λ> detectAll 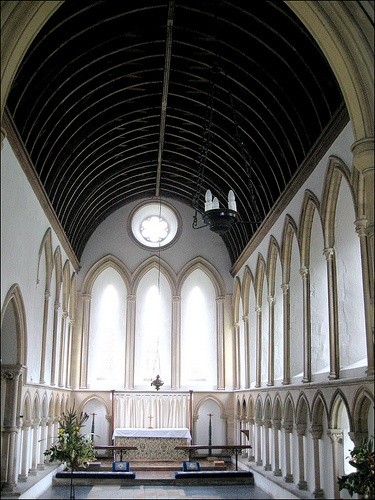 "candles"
[212,197,220,210]
[227,189,237,209]
[203,190,212,210]
